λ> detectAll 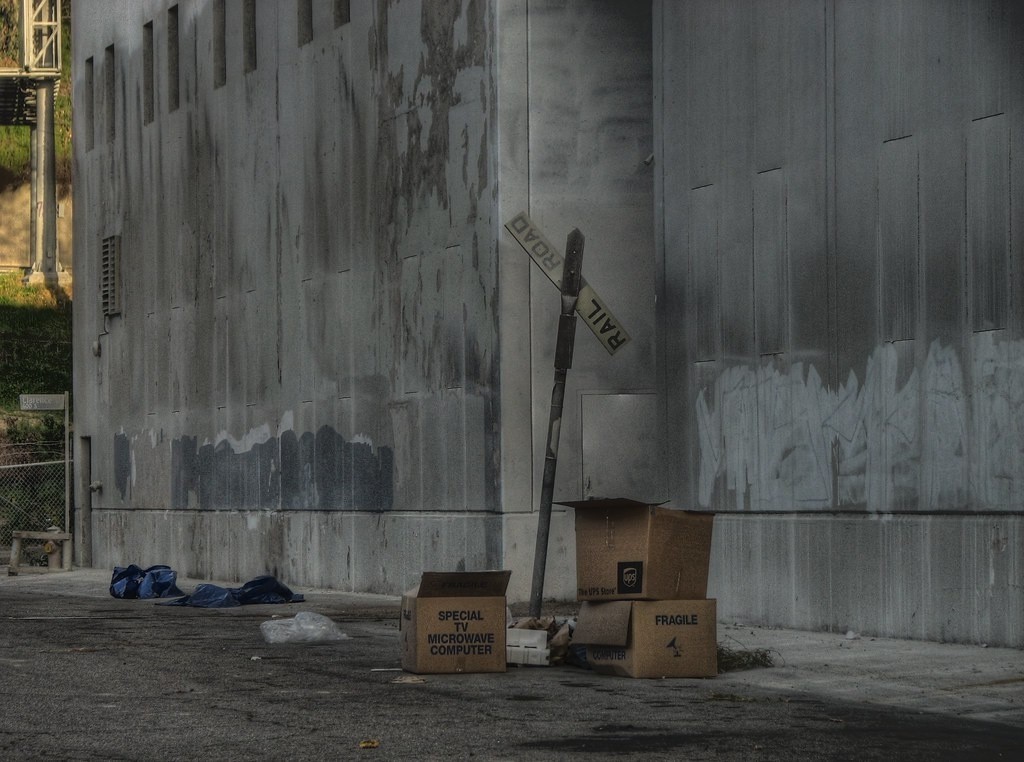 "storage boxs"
[567,598,719,678]
[550,497,716,600]
[398,571,513,673]
